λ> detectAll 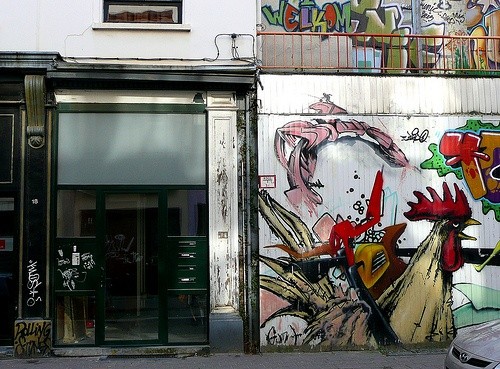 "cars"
[442,319,500,368]
